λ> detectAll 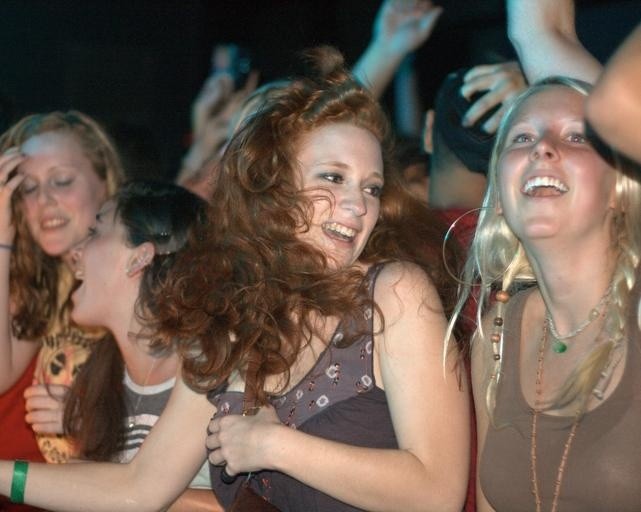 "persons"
[0,1,641,512]
